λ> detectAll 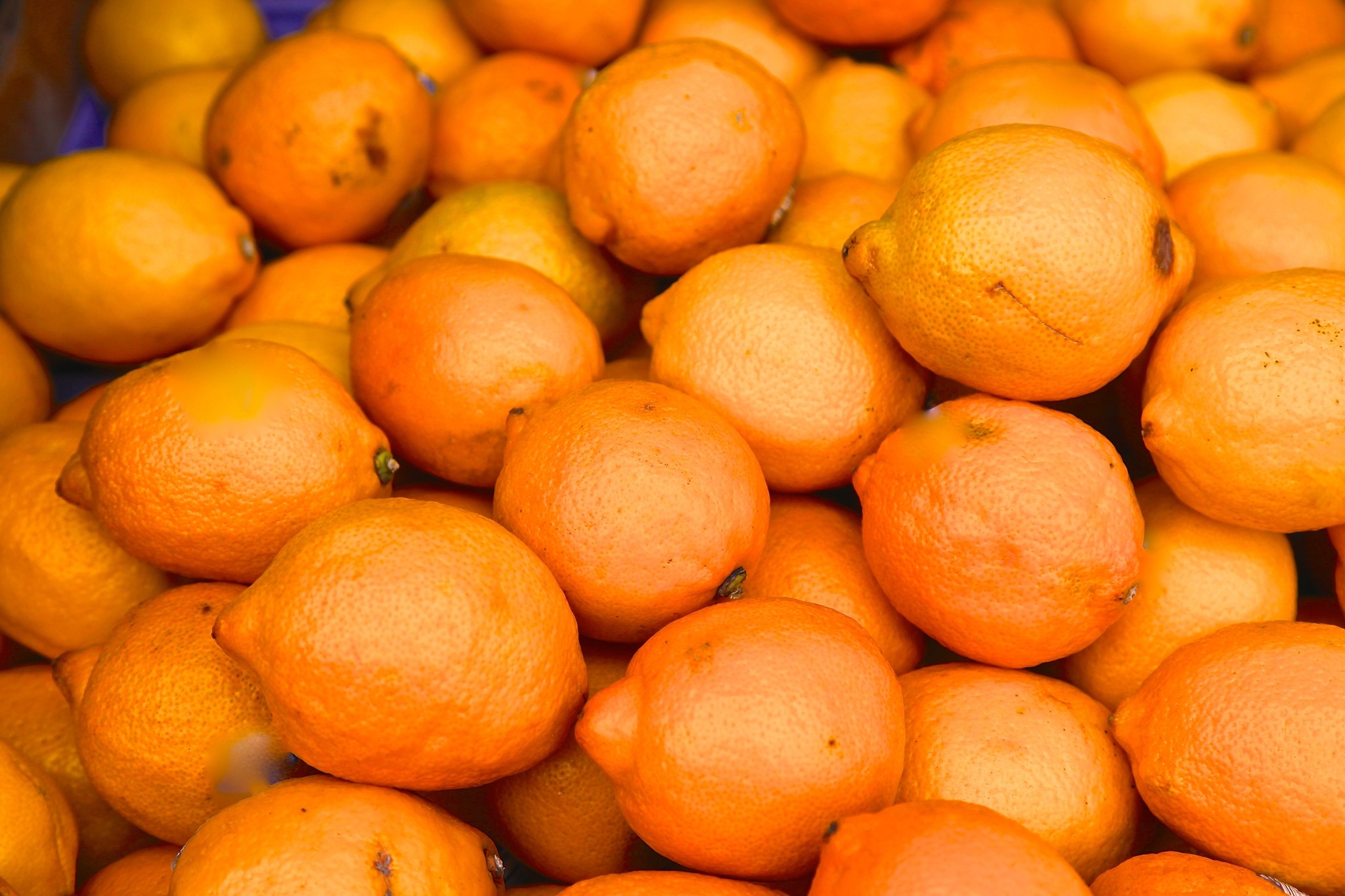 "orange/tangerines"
[0,1,1343,896]
